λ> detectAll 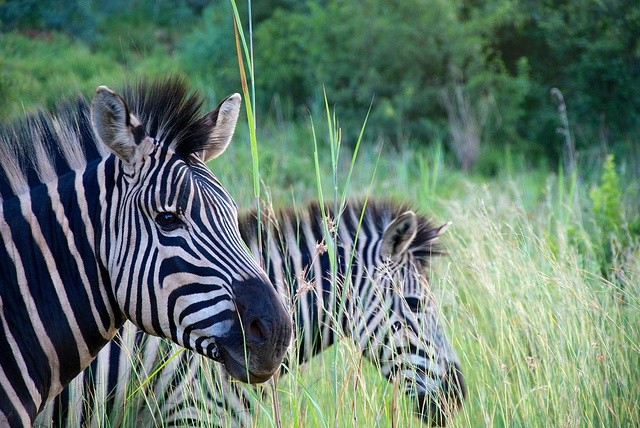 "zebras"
[33,197,466,428]
[0,71,293,428]
